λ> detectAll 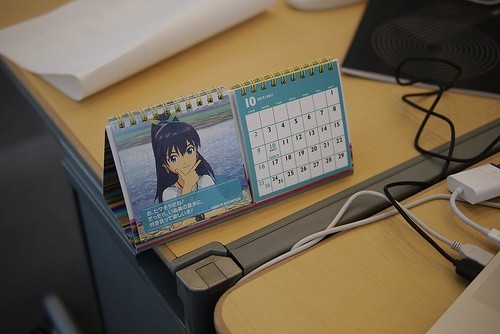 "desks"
[0,0,500,334]
